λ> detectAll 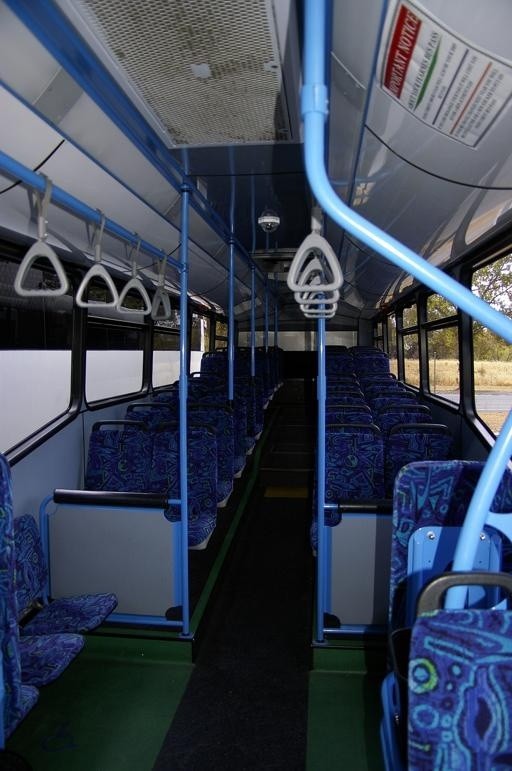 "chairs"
[312,346,512,769]
[15,514,118,637]
[87,346,282,557]
[1,461,83,748]
